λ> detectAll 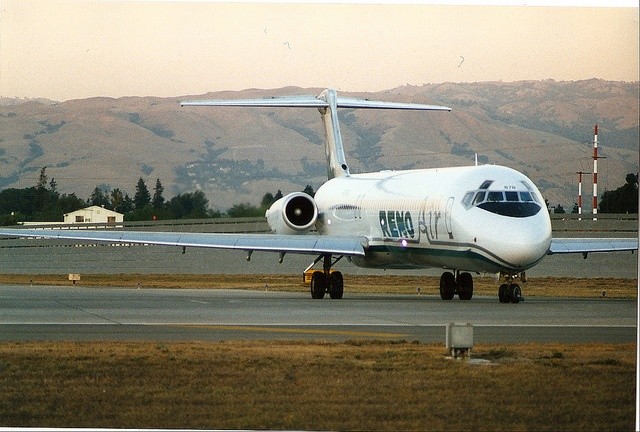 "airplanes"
[0,88,639,304]
